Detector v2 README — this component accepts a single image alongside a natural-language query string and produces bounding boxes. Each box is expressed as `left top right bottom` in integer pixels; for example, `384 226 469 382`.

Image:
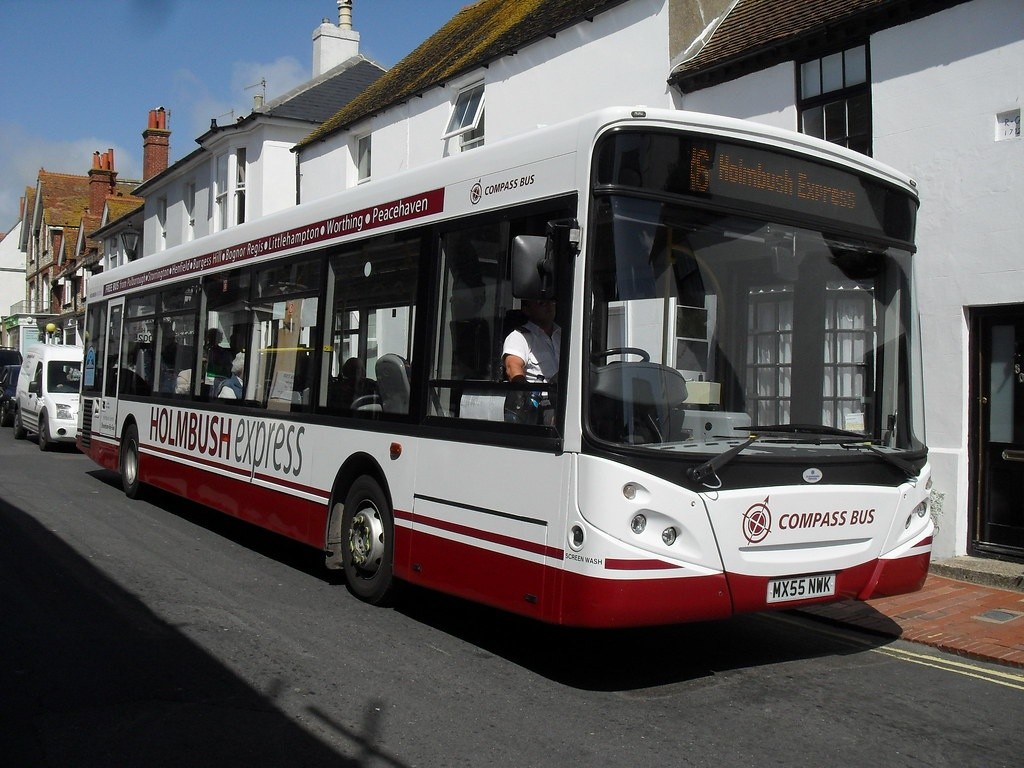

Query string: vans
14 342 86 453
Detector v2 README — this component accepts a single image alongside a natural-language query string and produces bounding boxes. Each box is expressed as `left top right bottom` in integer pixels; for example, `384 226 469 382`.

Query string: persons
337 358 377 405
501 300 562 427
39 364 63 396
175 369 192 394
204 328 223 348
214 352 244 399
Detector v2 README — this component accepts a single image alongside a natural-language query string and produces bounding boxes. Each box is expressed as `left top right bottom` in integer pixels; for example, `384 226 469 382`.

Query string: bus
76 107 939 629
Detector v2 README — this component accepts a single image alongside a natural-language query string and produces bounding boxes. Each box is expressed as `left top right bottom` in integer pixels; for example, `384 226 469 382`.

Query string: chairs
375 353 410 413
502 307 529 339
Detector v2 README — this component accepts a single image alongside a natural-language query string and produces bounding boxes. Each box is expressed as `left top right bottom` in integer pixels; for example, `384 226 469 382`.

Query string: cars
0 364 22 428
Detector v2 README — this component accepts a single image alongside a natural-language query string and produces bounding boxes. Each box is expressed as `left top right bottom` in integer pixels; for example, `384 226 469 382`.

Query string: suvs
0 345 23 377
